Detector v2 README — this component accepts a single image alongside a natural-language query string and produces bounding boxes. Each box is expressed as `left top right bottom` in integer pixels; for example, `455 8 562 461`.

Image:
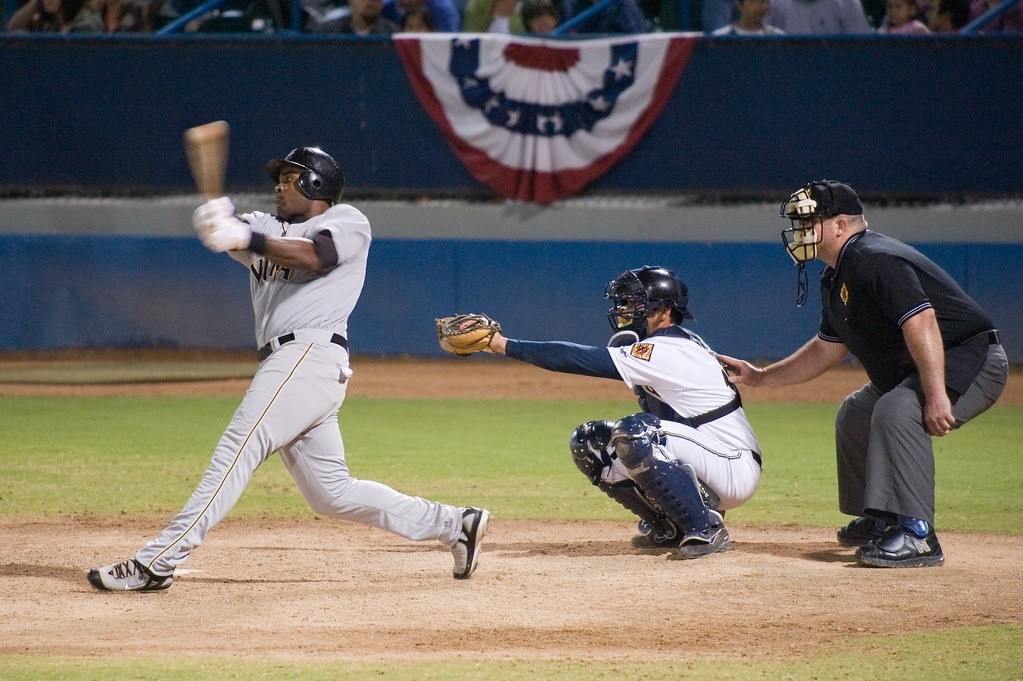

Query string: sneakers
836 516 897 546
681 527 732 559
88 560 174 591
452 508 493 580
631 530 683 548
856 526 947 567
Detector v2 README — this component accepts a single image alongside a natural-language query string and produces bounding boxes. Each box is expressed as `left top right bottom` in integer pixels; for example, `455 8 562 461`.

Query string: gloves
192 194 266 255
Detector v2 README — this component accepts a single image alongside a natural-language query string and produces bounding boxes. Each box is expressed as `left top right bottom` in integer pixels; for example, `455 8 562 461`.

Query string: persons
436 264 763 558
708 180 1008 567
7 0 1023 38
88 146 492 594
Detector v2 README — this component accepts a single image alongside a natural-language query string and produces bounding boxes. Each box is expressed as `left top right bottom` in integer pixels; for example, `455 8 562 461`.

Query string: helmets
267 146 347 205
629 266 694 318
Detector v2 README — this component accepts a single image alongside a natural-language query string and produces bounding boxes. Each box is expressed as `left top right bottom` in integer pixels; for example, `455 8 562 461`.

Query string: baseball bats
181 121 229 206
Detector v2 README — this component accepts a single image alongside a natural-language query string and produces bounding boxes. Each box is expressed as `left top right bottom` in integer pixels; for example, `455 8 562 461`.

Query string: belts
751 450 762 467
989 330 1001 345
257 330 348 362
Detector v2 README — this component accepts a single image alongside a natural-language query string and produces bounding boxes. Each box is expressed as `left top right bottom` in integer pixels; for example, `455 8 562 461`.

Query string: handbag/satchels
941 334 990 396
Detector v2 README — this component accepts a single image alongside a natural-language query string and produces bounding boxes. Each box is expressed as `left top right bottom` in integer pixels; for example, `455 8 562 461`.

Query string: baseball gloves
434 312 503 359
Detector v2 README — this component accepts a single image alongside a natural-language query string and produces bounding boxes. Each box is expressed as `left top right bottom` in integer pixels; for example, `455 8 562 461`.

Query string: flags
394 33 705 205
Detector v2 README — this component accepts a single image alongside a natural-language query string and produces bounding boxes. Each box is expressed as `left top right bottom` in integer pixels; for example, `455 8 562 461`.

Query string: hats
806 181 864 218
